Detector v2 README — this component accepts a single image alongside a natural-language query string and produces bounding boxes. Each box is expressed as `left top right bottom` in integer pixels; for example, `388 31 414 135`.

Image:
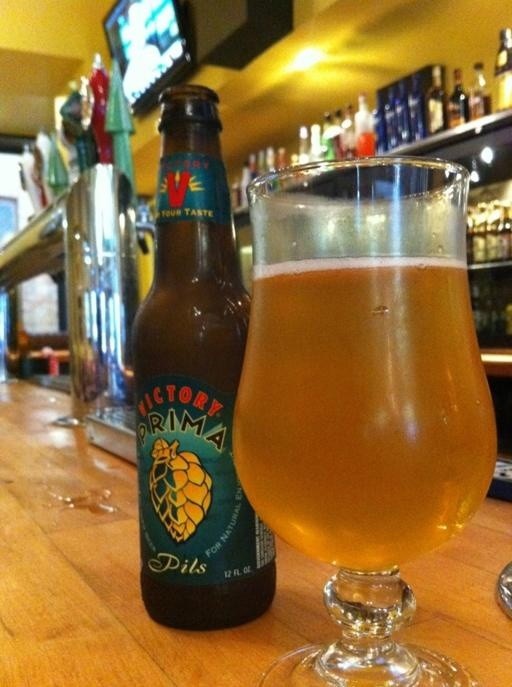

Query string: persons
121 1 163 103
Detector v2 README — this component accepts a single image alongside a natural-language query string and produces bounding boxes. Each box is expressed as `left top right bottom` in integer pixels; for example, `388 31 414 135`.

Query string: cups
60 159 144 425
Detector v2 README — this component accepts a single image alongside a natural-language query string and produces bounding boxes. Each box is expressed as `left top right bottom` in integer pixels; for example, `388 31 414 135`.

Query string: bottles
134 81 272 633
241 28 512 356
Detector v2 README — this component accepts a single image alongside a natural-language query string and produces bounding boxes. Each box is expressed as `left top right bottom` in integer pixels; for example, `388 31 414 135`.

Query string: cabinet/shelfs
232 107 512 376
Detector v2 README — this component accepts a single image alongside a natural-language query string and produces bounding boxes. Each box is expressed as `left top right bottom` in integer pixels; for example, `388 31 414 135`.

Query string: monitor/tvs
101 0 194 119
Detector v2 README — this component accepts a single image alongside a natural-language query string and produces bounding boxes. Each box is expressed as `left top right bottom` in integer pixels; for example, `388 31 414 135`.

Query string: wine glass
229 154 500 687
